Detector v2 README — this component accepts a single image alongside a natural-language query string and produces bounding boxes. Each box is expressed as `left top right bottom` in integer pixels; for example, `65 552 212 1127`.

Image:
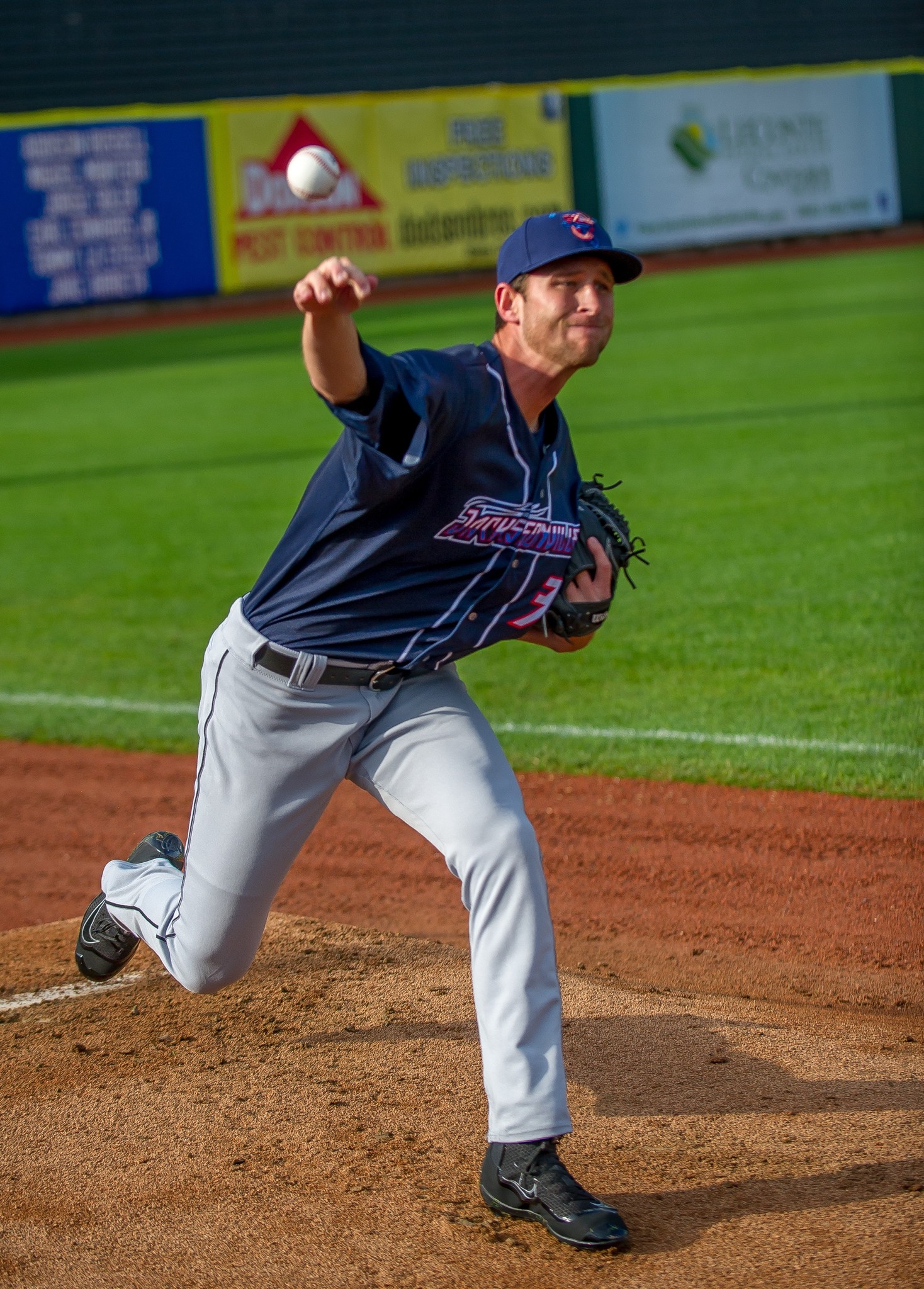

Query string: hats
495 211 643 286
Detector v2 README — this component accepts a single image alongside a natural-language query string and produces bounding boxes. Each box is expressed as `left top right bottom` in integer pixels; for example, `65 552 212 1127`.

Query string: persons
75 209 642 1247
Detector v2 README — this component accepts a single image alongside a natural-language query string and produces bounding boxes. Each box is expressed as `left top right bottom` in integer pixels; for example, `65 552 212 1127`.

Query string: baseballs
286 145 340 203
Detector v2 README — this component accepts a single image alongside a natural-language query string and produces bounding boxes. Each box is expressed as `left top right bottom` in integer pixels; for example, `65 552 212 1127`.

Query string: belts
253 644 408 693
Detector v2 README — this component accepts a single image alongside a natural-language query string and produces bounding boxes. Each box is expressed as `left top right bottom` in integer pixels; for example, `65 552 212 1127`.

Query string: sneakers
73 831 186 982
478 1138 628 1248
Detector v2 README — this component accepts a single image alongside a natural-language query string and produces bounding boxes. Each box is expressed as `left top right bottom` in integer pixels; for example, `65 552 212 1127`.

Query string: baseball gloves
530 480 634 639
